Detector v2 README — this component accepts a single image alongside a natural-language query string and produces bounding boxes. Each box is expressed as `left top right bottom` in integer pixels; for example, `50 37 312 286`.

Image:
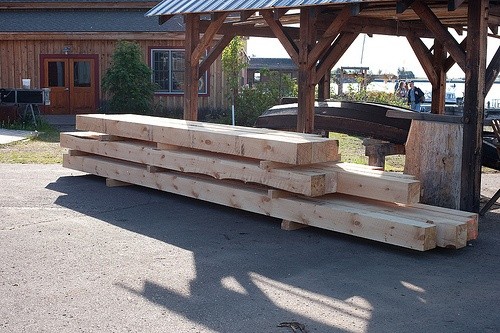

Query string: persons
404 82 410 97
396 81 406 97
408 80 424 111
